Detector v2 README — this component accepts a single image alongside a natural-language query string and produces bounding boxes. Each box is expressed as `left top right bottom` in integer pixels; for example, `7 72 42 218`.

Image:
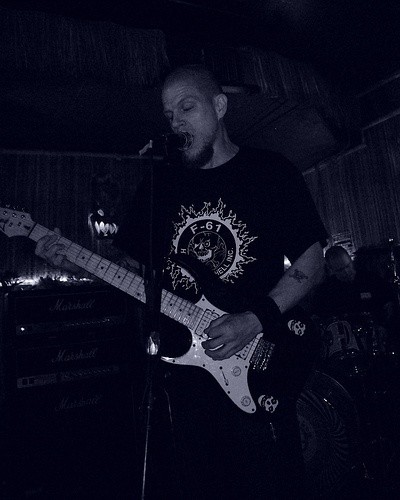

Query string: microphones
160 132 191 152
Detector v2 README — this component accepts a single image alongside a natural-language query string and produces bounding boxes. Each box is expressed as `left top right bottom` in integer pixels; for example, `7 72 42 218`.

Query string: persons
316 246 393 340
35 64 330 500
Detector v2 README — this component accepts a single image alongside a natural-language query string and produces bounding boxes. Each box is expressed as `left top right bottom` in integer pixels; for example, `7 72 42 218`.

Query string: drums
302 371 362 496
361 335 390 372
318 316 361 370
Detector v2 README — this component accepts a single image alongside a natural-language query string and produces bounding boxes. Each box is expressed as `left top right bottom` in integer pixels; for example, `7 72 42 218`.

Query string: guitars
0 204 315 423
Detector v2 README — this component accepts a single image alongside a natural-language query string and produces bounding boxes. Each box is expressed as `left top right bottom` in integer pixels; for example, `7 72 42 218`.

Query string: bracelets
245 292 282 334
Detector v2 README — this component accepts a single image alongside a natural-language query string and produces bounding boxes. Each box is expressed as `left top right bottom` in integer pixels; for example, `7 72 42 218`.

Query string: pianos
11 315 139 360
